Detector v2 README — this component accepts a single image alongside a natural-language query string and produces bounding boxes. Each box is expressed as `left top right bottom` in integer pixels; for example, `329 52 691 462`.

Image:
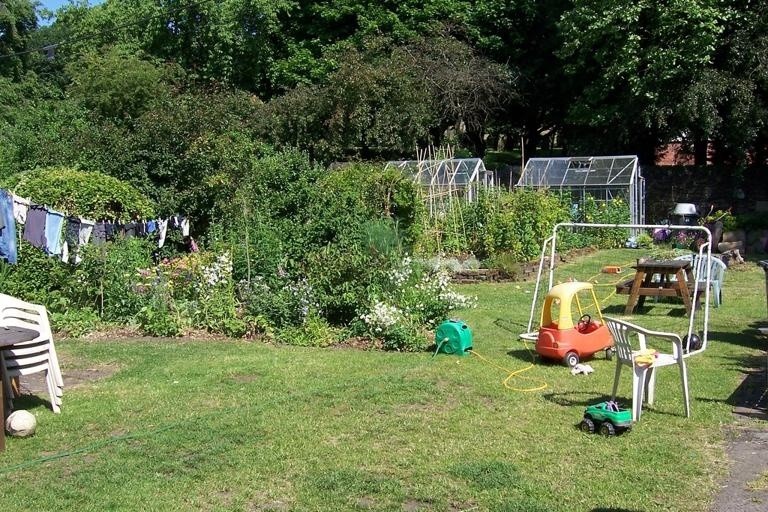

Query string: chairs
600 315 690 421
651 254 728 309
1 291 65 418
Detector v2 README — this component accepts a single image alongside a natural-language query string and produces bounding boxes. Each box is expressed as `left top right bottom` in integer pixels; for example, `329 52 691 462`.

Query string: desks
624 260 703 319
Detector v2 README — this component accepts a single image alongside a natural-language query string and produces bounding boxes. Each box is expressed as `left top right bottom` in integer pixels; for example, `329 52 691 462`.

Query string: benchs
615 278 712 314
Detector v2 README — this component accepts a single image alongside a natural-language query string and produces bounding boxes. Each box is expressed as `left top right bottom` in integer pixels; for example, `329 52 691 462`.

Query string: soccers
683 333 701 350
